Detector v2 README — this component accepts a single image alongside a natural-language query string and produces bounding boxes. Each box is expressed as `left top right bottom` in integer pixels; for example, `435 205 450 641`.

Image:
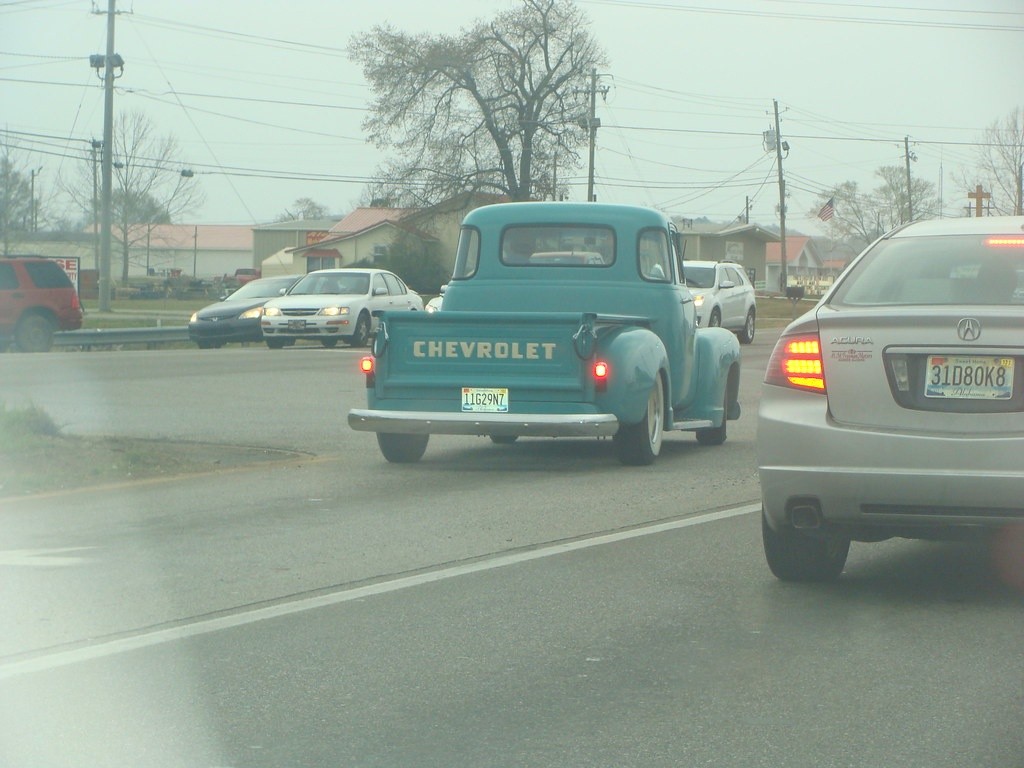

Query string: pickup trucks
347 201 742 467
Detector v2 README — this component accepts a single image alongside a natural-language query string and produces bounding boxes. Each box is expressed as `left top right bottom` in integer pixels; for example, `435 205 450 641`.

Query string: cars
262 267 423 349
188 274 304 347
424 284 448 313
755 215 1024 589
1 251 85 353
231 268 260 290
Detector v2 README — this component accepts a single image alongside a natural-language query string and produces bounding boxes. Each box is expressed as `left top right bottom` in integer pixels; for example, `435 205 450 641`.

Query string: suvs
667 259 759 344
